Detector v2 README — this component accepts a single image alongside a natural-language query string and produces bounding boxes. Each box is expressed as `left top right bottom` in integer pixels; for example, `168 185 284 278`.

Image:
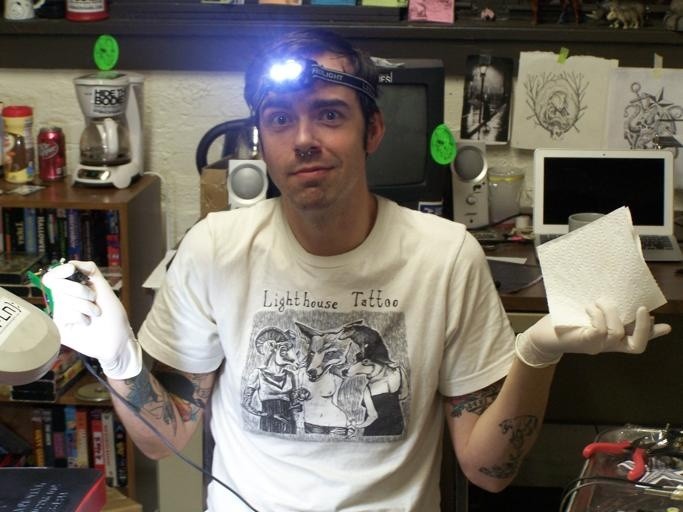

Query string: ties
269 57 447 219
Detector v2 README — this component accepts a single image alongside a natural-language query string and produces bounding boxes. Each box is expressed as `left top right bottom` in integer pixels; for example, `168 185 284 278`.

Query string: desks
141 208 682 512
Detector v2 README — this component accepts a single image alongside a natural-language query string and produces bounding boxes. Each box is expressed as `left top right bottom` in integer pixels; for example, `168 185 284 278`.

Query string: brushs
72 69 146 191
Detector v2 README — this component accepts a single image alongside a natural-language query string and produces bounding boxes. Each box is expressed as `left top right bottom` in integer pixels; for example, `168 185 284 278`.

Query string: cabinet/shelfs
0 173 164 512
0 104 35 183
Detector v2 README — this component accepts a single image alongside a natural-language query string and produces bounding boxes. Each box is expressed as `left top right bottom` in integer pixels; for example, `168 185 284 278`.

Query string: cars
568 212 604 233
487 161 526 225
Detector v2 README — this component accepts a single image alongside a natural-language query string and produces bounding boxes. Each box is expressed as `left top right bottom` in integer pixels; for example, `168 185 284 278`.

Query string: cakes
38 127 65 182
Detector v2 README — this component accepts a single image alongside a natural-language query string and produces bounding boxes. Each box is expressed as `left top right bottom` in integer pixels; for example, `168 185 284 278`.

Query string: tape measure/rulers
582 436 656 480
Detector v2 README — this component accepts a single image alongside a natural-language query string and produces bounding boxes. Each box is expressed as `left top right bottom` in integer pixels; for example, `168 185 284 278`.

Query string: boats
451 139 490 230
227 158 270 210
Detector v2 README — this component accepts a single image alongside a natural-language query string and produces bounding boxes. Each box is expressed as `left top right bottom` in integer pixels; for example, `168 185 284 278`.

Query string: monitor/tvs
0 208 129 494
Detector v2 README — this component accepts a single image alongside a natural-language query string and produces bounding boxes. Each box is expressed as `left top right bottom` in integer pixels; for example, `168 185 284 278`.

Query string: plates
0 173 164 512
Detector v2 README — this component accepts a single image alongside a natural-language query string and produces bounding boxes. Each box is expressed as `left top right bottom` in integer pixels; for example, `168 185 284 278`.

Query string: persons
37 25 674 512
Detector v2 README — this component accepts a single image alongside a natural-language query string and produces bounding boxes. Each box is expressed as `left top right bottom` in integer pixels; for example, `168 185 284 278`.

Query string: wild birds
533 149 682 263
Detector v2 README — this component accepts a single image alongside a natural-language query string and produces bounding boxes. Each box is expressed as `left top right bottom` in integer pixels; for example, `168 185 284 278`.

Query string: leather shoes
514 301 672 369
41 259 144 380
